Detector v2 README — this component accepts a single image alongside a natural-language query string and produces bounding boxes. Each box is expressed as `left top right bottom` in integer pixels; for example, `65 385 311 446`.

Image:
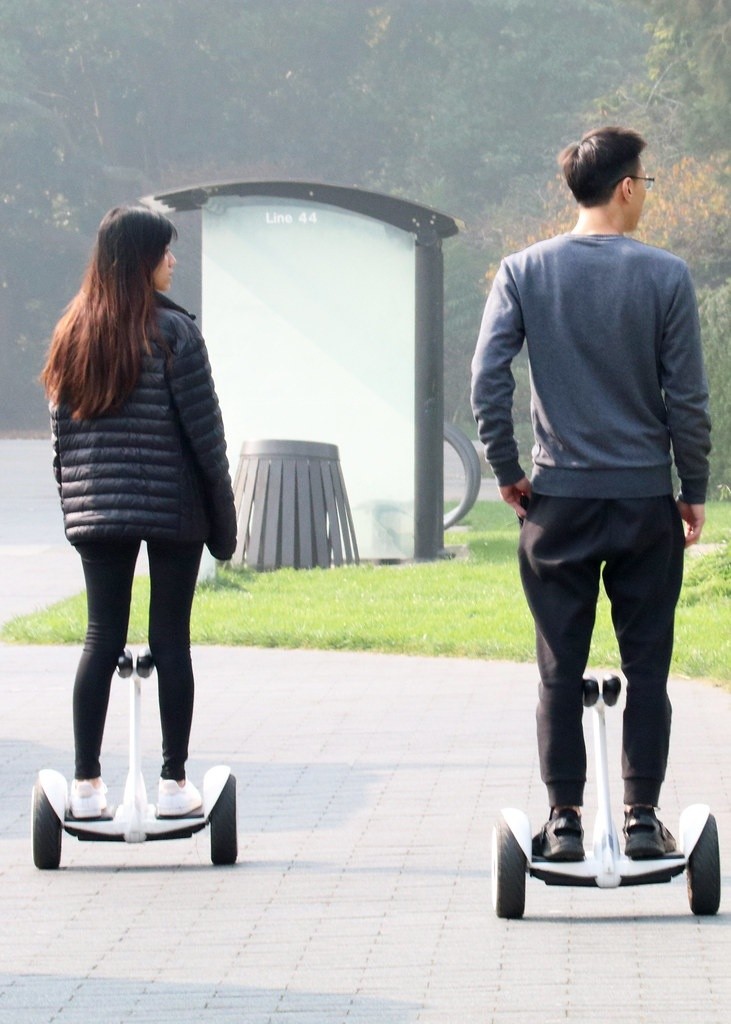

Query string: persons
467 125 716 858
39 204 239 821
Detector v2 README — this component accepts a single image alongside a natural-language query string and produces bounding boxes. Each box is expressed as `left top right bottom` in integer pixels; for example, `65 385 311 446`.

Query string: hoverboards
29 650 237 866
495 674 720 921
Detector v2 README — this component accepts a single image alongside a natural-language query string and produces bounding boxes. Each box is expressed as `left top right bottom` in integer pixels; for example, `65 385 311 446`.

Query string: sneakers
70 778 108 817
532 809 585 861
622 805 677 856
158 777 203 815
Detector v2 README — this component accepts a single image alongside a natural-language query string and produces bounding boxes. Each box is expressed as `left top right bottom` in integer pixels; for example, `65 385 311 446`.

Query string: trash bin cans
221 439 360 572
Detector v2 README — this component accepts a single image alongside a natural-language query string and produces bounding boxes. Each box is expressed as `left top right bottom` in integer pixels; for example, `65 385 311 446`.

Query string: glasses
618 174 655 191
